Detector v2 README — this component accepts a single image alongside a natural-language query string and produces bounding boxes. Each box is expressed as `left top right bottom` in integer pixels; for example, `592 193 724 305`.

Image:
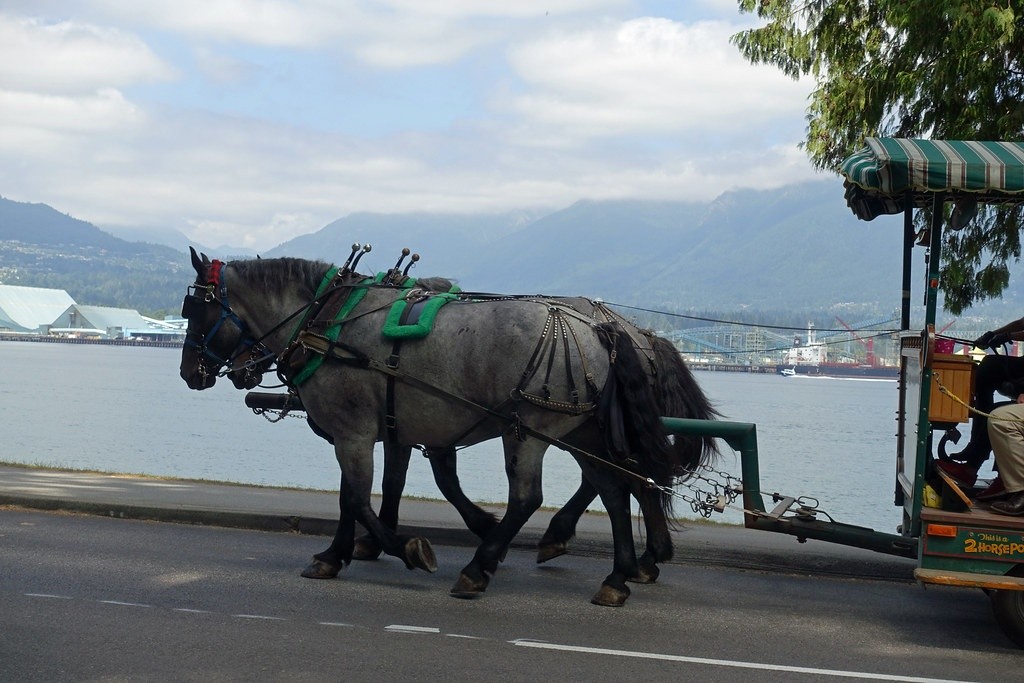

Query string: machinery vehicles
832 313 879 370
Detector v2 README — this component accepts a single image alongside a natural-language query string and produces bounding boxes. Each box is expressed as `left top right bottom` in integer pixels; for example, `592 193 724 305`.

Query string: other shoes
949 442 981 461
974 477 1007 498
990 493 1024 515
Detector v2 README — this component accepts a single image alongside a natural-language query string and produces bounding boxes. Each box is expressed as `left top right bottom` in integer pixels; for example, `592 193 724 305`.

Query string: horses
180 245 721 607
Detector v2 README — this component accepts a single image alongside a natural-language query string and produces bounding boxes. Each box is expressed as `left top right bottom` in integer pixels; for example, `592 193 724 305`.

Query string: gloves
972 330 994 350
988 331 1013 348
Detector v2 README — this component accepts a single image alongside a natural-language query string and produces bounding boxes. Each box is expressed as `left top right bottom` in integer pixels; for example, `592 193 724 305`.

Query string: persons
935 318 1024 516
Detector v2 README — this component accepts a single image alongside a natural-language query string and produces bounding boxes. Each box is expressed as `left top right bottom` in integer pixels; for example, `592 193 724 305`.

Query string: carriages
176 136 1024 647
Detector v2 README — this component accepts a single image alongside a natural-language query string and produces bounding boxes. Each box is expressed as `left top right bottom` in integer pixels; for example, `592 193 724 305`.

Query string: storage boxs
928 353 978 423
